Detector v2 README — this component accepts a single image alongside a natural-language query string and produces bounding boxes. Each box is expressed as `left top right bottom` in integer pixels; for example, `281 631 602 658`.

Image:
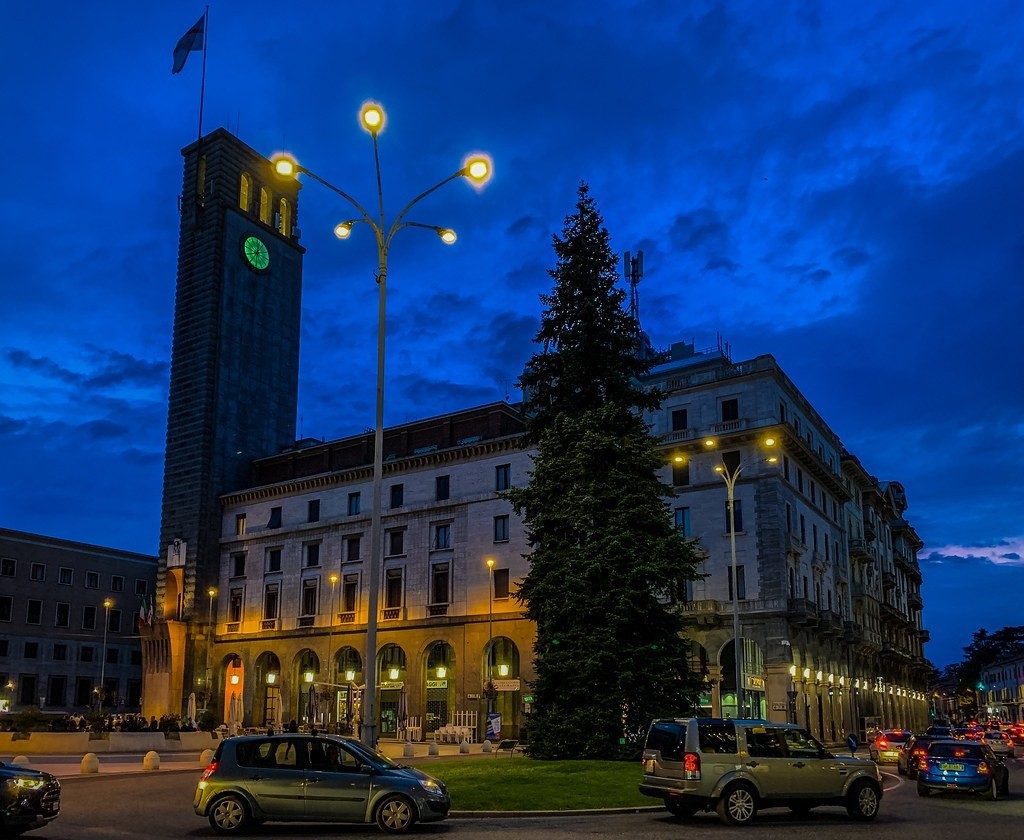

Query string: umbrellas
187 690 196 722
274 694 282 734
345 682 355 734
228 691 236 735
237 693 244 729
306 683 317 720
396 686 408 740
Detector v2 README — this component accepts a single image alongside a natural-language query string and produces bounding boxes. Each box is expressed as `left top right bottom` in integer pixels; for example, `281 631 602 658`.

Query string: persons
307 720 352 735
320 745 341 772
268 725 275 736
47 697 228 733
289 720 298 733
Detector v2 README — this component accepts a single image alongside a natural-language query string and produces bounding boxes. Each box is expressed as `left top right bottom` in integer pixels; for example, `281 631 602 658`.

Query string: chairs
434 726 471 744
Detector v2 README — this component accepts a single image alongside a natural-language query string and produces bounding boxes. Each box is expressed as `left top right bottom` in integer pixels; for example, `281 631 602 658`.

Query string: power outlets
266 655 276 684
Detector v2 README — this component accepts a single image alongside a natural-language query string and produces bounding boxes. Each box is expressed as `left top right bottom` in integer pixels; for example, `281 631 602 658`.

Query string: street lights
203 591 215 708
97 602 112 712
265 100 495 747
486 559 494 684
327 575 338 690
669 433 783 719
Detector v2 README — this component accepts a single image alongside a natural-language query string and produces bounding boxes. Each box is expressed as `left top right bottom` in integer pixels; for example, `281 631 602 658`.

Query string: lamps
198 668 211 685
496 637 510 676
388 644 399 679
302 650 314 682
435 640 447 677
229 656 239 685
345 646 355 681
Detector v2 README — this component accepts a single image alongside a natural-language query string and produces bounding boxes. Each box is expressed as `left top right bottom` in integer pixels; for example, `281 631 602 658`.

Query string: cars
0 761 63 840
192 731 452 836
868 729 914 766
916 738 1009 802
896 709 1024 780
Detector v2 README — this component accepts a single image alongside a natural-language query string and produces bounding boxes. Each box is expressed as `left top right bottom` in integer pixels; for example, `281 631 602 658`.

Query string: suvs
637 717 884 827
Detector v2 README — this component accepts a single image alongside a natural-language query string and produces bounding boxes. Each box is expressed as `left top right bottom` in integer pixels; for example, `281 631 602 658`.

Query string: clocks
239 232 273 276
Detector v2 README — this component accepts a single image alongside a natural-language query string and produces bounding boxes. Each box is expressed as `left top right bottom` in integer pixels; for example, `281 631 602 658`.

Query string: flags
172 14 205 74
138 599 148 628
147 596 153 627
